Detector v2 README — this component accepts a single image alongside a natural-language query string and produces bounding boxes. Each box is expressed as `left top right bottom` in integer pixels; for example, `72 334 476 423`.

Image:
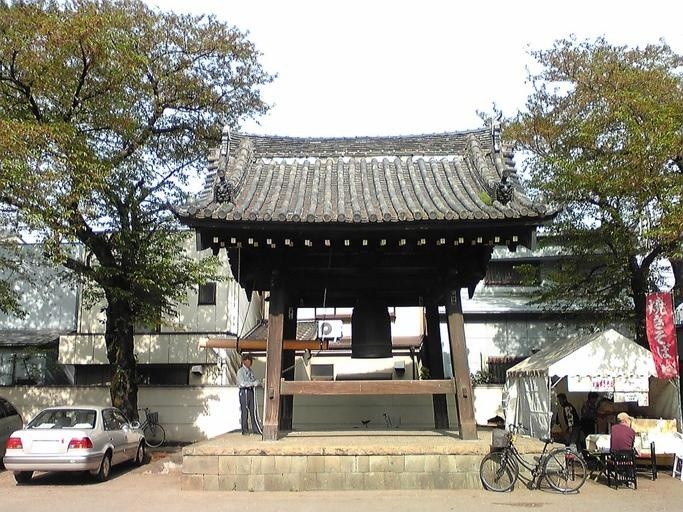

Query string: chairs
606 448 637 490
77 414 95 426
55 417 71 427
636 441 657 481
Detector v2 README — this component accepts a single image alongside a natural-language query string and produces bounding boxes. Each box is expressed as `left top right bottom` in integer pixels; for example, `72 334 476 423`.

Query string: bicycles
137 406 165 449
351 411 402 430
480 422 589 496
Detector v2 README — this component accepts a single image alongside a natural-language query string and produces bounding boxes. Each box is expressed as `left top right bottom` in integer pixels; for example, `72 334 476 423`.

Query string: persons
572 391 627 470
610 412 635 488
237 354 262 435
552 393 580 446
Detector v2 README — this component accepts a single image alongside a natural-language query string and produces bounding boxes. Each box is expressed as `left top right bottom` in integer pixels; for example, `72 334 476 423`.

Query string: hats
617 413 633 422
242 354 255 361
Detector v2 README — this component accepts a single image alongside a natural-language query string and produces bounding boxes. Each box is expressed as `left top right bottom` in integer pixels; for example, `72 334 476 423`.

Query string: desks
583 449 637 482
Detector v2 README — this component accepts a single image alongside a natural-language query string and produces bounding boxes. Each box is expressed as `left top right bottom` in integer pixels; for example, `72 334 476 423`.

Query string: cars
2 404 147 482
0 395 23 460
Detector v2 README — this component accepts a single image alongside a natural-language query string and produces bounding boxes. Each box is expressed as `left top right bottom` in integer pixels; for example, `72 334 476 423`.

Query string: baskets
492 428 510 448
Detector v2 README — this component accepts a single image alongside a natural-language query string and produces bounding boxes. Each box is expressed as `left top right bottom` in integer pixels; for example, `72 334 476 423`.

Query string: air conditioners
318 320 343 338
